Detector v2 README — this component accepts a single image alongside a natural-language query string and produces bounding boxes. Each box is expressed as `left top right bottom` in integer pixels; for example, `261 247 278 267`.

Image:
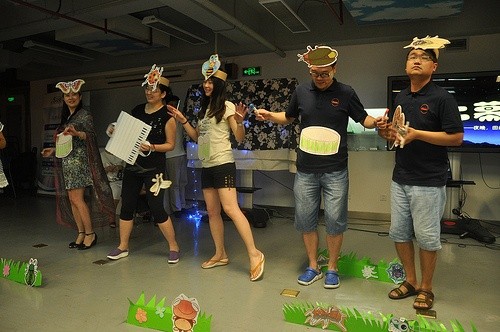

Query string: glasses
407 53 437 63
310 68 332 77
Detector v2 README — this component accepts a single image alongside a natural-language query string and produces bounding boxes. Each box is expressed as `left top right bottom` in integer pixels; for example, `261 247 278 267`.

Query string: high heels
69 232 85 248
77 232 97 250
250 253 265 281
202 258 229 268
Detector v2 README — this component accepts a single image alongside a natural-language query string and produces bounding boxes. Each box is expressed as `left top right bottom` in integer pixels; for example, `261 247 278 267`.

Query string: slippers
388 281 420 299
297 268 323 286
325 271 340 288
413 290 434 309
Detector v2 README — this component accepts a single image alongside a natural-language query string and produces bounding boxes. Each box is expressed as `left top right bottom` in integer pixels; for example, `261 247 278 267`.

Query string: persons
378 49 464 310
105 77 180 263
166 67 265 281
0 121 9 188
254 43 389 288
41 90 116 250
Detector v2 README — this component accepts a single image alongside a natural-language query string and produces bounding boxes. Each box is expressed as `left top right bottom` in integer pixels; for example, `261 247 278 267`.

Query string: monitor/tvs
387 70 500 153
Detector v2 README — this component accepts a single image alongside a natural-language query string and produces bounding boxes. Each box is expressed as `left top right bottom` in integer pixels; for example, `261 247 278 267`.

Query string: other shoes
107 248 128 259
167 250 180 264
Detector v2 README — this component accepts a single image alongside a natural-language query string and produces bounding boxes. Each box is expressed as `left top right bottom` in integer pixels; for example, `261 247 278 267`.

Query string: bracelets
182 121 187 125
